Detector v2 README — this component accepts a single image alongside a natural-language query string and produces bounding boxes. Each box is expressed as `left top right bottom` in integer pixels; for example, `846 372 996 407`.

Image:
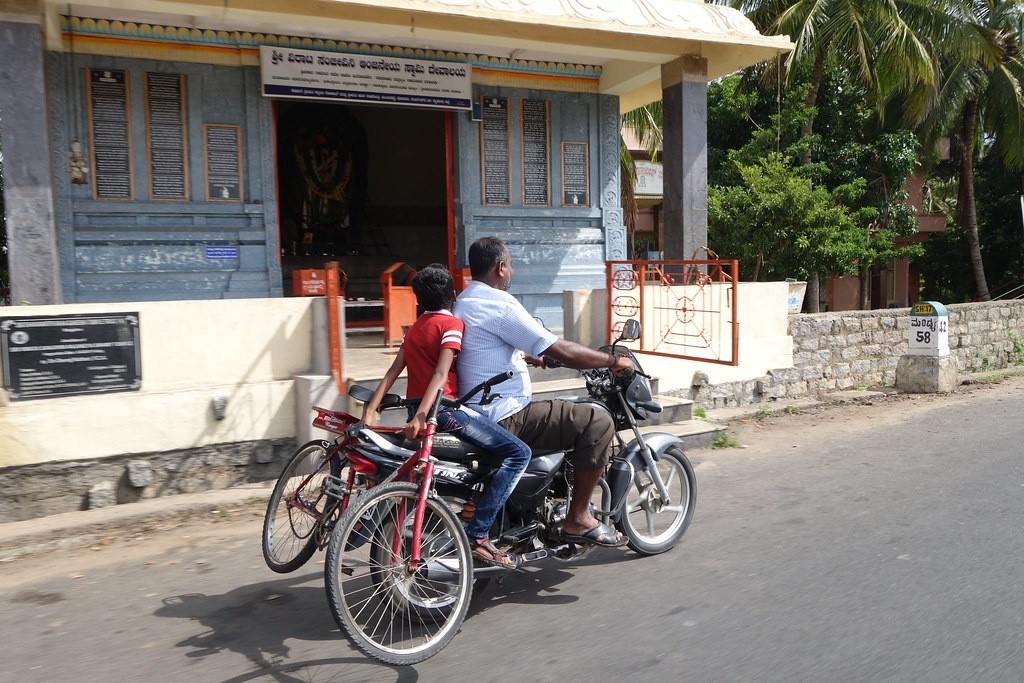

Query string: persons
450 236 637 548
351 262 532 571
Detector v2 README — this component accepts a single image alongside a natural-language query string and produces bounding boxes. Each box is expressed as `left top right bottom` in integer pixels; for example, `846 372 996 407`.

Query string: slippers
563 517 629 546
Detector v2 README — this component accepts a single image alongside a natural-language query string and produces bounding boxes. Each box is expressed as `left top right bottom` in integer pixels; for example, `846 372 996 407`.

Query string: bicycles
262 370 515 665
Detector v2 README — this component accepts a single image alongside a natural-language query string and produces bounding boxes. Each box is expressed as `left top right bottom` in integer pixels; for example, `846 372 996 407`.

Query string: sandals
464 530 516 569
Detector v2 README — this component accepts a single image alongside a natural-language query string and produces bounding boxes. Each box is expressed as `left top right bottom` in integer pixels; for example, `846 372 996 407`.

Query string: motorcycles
340 318 698 625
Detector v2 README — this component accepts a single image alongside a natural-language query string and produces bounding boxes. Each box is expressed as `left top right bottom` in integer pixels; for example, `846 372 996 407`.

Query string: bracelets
611 355 617 368
